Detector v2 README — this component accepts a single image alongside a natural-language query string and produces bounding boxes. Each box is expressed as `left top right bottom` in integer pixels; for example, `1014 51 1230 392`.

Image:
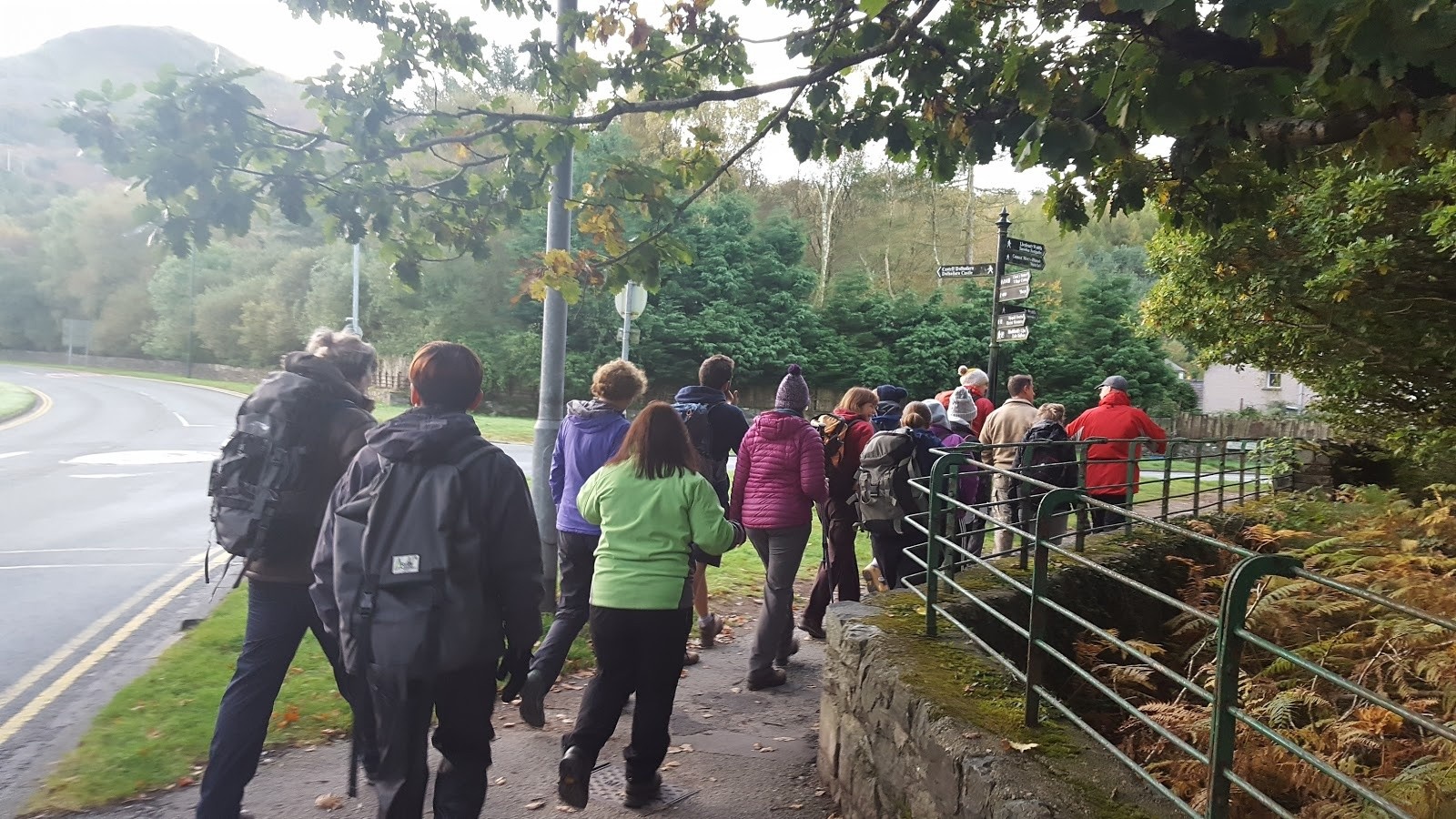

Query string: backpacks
810 412 862 477
855 429 916 535
205 371 325 559
1022 431 1071 506
935 433 979 510
333 433 505 698
667 400 715 486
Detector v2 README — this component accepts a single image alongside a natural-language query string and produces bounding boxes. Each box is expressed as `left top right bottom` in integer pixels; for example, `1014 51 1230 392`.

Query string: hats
921 399 952 431
946 387 978 421
775 364 810 410
958 365 990 386
1093 376 1127 393
876 385 907 402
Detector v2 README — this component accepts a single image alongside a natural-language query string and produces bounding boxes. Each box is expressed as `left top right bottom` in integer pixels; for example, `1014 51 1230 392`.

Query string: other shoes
519 669 552 727
697 614 724 648
797 617 826 639
683 651 699 666
558 745 594 809
747 667 788 691
622 772 665 808
776 639 800 666
862 564 886 593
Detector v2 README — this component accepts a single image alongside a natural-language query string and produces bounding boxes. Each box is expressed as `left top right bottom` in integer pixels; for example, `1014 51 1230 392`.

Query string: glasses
1098 386 1112 391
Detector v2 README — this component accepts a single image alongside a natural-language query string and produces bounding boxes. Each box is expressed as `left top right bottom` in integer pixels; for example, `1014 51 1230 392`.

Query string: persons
310 340 544 819
193 333 377 819
517 362 1172 810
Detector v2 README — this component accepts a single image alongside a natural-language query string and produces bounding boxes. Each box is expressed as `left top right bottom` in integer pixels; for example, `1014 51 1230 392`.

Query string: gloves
728 518 747 546
497 654 530 703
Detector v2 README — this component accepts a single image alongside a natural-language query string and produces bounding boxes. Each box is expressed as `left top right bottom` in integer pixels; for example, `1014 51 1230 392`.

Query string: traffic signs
1000 304 1041 321
998 269 1032 303
937 263 995 278
994 311 1031 344
1003 238 1046 270
